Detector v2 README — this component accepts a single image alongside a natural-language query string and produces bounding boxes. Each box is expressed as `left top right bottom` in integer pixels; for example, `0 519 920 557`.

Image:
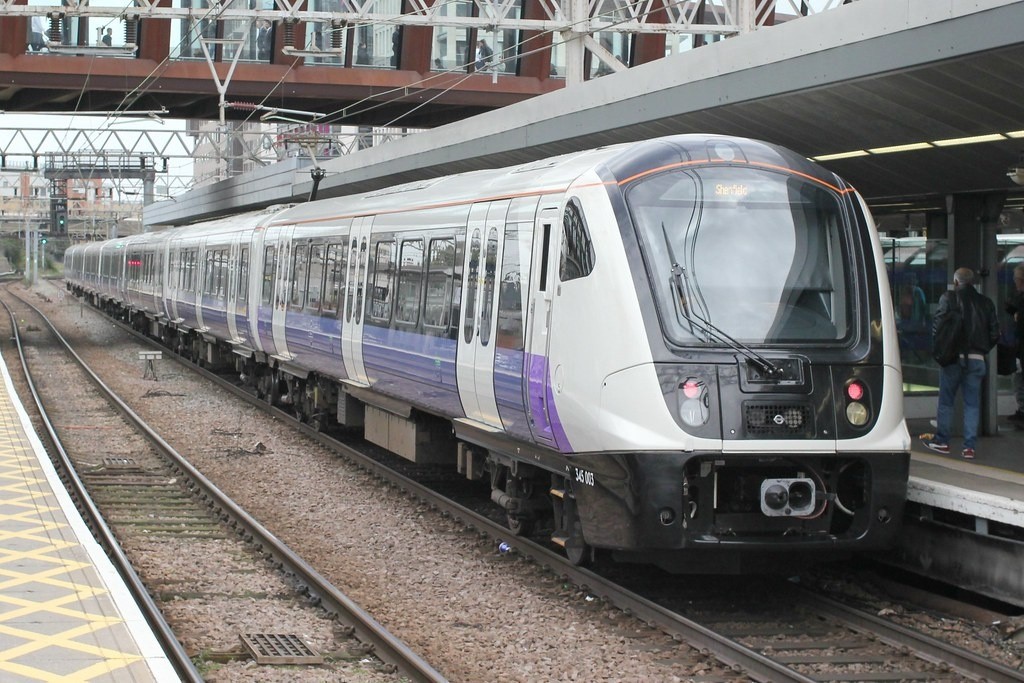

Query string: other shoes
1008 411 1024 420
1016 422 1024 430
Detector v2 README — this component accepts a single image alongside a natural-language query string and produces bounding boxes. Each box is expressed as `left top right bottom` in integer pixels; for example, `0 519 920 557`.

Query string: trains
62 132 910 587
876 235 1024 368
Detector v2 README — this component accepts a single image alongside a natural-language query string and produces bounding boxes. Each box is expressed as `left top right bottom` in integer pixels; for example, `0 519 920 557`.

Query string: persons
256 19 272 60
354 24 622 77
102 28 112 45
915 267 1001 460
1004 264 1024 429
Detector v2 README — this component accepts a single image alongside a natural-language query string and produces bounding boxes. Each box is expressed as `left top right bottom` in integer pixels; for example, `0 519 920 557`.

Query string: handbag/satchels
929 290 968 366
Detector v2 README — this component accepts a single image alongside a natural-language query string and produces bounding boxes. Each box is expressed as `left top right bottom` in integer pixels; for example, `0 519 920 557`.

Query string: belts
958 354 986 362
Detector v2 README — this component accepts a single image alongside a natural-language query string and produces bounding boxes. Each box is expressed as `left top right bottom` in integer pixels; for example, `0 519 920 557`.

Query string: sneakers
961 448 975 458
919 433 950 454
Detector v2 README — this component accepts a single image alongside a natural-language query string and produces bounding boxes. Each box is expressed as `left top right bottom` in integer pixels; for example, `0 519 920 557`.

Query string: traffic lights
42 237 48 244
58 213 68 234
